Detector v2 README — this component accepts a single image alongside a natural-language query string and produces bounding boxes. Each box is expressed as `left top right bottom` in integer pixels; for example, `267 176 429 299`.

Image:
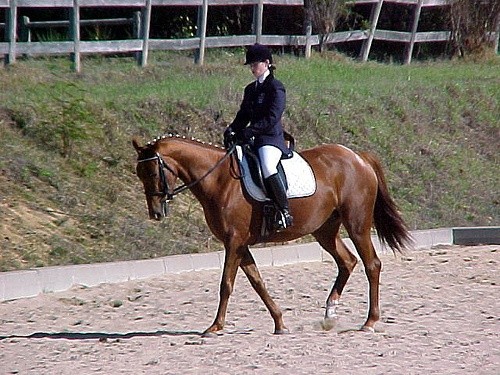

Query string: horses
131 133 415 338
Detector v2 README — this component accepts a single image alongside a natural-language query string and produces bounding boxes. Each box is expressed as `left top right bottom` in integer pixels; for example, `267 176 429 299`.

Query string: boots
263 172 293 229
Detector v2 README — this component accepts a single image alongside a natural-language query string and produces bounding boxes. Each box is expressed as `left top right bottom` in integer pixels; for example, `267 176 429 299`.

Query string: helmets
243 42 273 66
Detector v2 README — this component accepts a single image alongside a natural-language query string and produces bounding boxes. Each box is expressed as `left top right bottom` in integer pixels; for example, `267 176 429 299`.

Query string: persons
224 43 294 229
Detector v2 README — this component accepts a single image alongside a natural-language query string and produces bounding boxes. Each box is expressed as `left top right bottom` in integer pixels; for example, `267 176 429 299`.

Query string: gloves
233 127 254 146
223 128 233 150
